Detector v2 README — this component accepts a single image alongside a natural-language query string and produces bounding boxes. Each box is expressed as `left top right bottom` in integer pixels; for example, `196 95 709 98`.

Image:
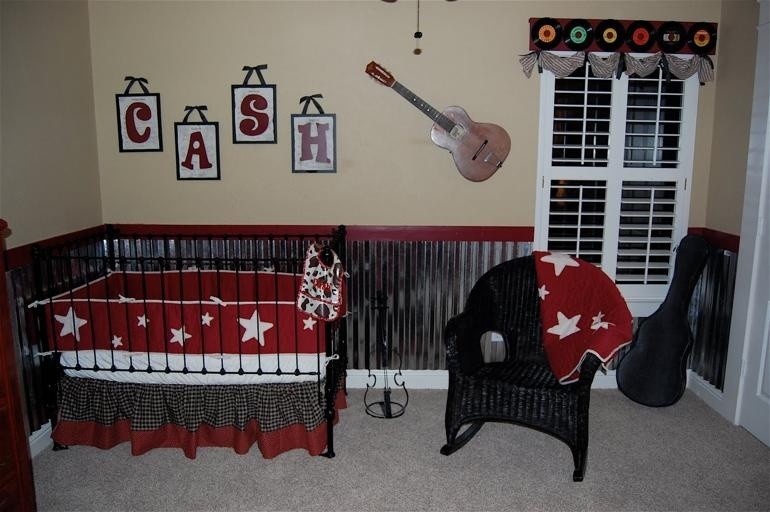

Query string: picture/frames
291 112 338 175
114 91 159 152
230 83 277 144
172 120 221 181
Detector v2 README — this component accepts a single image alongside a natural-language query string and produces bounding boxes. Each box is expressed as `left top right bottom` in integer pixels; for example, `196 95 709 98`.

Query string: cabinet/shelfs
0 218 40 512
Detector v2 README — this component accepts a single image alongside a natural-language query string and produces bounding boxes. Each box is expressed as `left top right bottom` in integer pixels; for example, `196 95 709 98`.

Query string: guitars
364 59 511 182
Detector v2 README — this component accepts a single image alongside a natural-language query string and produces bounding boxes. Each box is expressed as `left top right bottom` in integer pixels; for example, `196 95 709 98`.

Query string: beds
19 218 349 463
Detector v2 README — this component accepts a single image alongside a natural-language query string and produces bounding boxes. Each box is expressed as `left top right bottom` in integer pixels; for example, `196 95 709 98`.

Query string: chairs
438 251 630 482
